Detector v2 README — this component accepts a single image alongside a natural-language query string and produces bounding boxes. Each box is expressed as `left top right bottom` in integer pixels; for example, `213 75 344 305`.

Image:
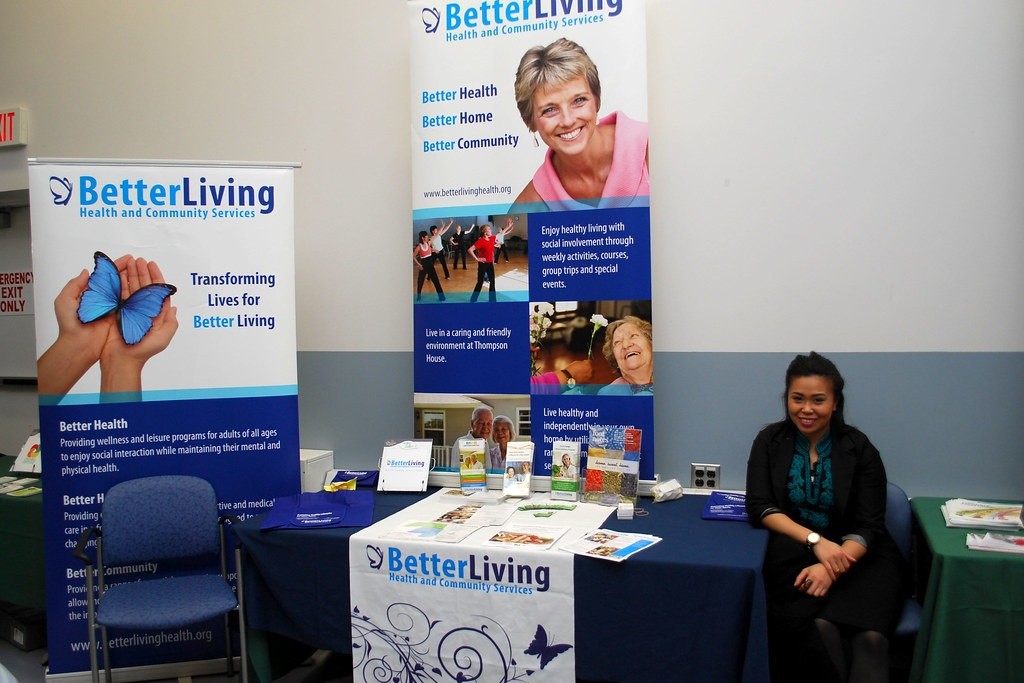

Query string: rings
803 580 812 588
835 569 839 572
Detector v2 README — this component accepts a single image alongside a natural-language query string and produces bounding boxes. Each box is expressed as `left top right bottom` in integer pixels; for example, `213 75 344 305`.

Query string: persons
468 219 514 303
507 462 530 482
36 255 179 405
413 219 454 302
597 315 655 395
450 223 476 269
489 415 516 470
560 454 577 479
745 351 909 683
530 360 594 395
450 406 493 474
508 38 649 215
471 453 483 469
460 457 472 470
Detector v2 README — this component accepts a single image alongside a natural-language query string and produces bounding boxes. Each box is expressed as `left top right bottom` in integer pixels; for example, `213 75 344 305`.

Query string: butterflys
75 250 177 346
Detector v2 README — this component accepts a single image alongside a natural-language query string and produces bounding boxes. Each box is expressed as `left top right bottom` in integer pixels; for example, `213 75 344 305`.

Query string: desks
907 496 1024 683
231 485 770 683
0 454 46 613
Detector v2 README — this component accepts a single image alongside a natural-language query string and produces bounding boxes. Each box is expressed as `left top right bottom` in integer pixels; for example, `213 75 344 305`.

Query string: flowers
588 313 609 360
529 302 555 375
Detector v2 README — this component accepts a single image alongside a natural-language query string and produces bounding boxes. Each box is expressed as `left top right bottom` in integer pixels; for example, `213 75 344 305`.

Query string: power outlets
690 463 721 490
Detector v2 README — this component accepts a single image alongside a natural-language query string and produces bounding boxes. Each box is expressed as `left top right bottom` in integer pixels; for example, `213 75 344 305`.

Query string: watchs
561 370 576 389
806 531 822 549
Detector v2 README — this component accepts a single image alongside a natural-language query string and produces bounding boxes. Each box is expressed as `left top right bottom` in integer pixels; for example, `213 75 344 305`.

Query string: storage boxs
0 601 47 652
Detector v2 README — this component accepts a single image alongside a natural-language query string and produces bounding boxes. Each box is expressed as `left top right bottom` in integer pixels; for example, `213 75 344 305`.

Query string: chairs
442 240 456 260
73 474 248 683
885 483 923 638
